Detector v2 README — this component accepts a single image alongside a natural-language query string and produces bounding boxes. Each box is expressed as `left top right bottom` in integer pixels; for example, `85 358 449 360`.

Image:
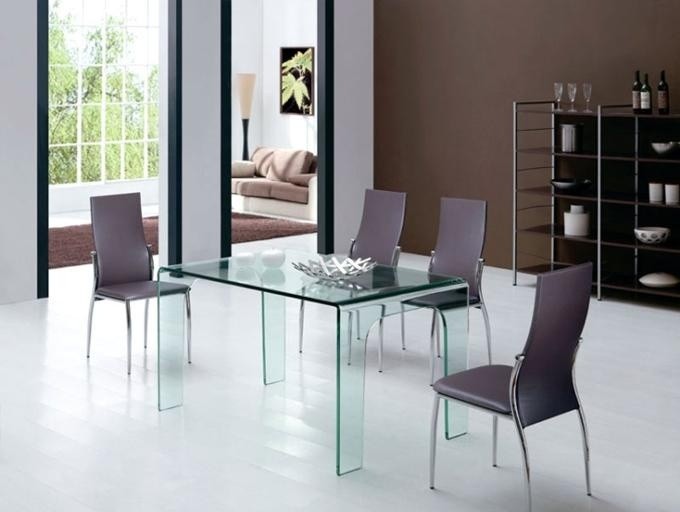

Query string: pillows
288 174 316 186
266 150 313 182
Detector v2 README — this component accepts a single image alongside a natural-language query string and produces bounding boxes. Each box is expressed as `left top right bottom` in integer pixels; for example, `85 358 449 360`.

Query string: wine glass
552 81 592 114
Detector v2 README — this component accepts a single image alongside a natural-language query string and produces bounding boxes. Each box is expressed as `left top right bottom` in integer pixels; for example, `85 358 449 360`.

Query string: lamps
234 71 258 162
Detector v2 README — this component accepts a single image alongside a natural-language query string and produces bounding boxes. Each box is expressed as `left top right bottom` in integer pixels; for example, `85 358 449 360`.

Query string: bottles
630 69 670 115
563 204 591 237
559 123 577 153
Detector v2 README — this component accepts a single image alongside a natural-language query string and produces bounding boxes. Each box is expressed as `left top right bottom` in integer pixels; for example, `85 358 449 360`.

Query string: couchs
231 147 317 226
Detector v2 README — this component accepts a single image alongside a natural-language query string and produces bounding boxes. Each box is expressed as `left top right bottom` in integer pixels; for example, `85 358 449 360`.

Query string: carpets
48 210 318 269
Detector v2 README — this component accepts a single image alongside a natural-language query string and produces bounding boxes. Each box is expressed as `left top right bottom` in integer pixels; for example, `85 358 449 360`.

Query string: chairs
422 259 601 512
296 186 410 375
398 194 496 387
80 188 196 376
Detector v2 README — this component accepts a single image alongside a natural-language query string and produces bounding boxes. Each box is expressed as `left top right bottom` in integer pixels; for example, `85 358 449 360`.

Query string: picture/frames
279 46 315 115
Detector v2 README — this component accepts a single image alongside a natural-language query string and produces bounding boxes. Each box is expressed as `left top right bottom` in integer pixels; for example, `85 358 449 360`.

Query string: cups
648 182 662 202
664 183 679 204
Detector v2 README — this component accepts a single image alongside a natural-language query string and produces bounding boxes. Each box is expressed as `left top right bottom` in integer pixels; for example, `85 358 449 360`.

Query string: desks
148 249 472 475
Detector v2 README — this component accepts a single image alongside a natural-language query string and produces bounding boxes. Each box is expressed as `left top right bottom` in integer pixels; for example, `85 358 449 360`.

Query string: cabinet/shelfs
516 96 679 304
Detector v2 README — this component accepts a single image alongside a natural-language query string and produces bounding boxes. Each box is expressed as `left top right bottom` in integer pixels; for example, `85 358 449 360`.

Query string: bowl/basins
550 178 592 197
651 142 680 154
634 226 670 244
